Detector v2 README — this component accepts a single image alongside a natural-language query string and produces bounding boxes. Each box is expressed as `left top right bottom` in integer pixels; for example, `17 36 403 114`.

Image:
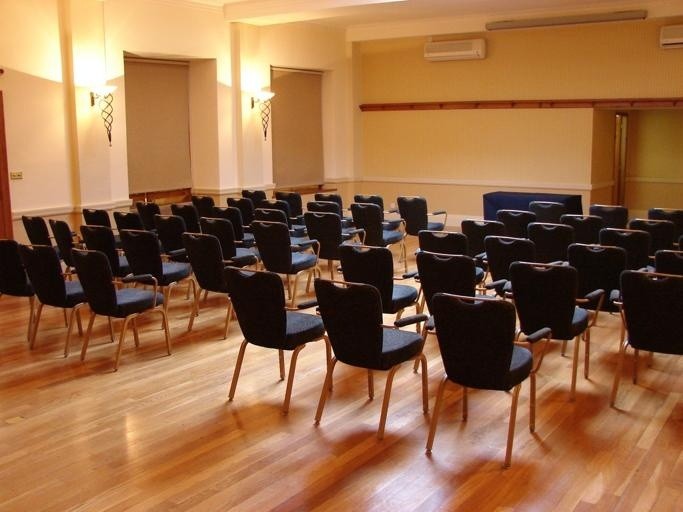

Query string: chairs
20 245 115 359
70 248 172 369
315 274 429 441
2 239 68 341
337 199 683 409
424 292 552 467
22 190 449 340
225 265 334 416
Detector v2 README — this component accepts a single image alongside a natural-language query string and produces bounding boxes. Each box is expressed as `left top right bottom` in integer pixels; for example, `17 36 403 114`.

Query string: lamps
251 90 276 140
90 85 118 146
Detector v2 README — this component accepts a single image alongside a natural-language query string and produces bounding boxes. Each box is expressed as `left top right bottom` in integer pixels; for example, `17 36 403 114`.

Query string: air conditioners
659 24 683 48
423 37 486 61
